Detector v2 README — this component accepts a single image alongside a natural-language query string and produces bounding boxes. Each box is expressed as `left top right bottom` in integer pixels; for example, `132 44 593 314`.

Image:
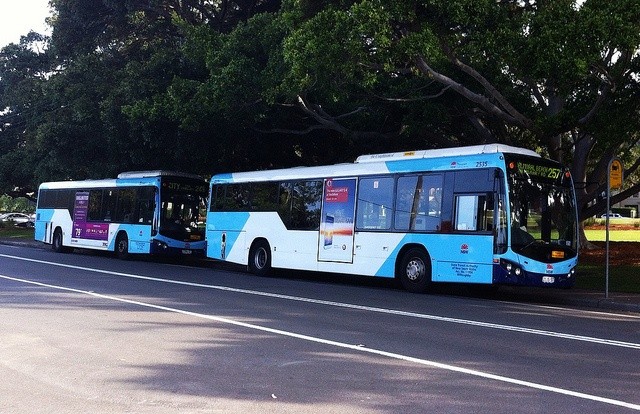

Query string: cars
601 212 627 218
1 213 35 227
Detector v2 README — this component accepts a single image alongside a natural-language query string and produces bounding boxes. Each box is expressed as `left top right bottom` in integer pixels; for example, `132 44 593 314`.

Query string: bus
35 170 211 261
203 144 579 294
